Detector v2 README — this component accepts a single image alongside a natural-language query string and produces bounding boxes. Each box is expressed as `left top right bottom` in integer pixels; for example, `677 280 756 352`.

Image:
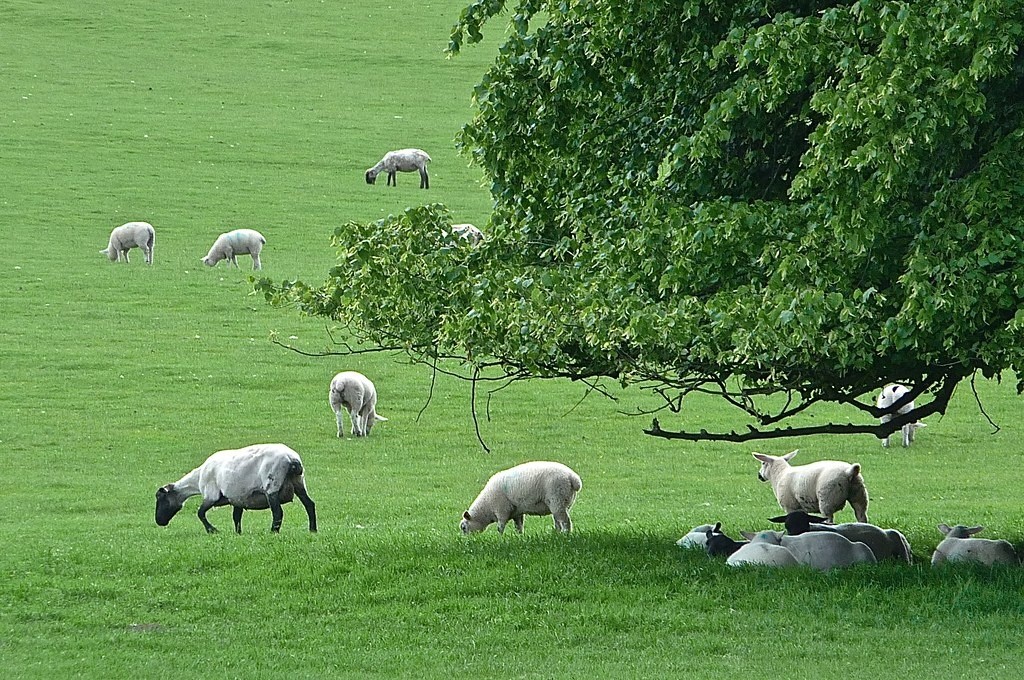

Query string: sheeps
155 443 318 535
876 384 927 448
672 510 1022 570
751 449 869 525
365 148 432 190
99 221 156 266
199 229 266 271
429 223 486 251
328 370 389 437
458 460 582 535
